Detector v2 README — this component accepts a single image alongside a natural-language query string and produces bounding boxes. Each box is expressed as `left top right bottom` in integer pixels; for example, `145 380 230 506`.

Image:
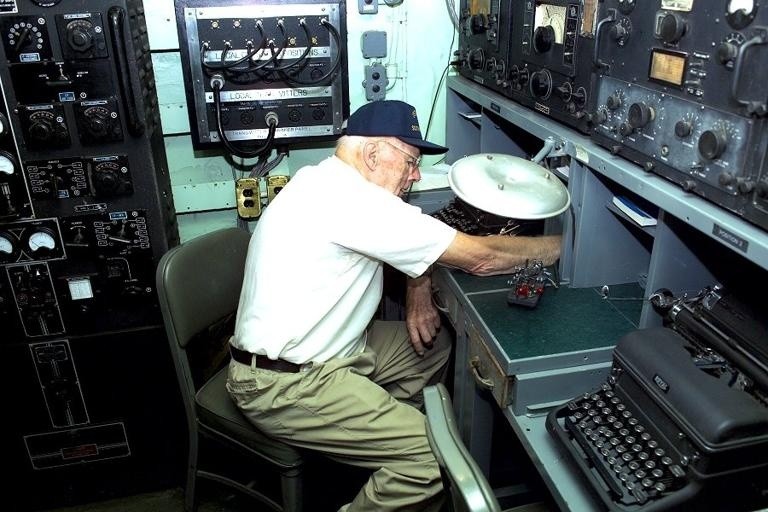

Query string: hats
340 97 449 156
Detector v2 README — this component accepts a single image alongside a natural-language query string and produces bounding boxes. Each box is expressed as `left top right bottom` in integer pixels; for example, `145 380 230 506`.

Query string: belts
227 339 303 376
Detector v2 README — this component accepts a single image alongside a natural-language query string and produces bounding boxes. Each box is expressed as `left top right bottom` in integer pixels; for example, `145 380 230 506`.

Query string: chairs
156 226 312 512
423 382 546 512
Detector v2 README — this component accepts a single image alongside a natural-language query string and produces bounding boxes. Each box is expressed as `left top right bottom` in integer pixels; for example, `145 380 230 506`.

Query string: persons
225 99 566 511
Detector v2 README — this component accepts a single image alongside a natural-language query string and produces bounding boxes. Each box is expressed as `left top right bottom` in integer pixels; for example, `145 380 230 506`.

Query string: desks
380 190 645 512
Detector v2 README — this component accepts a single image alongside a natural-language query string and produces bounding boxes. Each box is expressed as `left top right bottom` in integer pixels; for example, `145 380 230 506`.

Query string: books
611 196 659 228
